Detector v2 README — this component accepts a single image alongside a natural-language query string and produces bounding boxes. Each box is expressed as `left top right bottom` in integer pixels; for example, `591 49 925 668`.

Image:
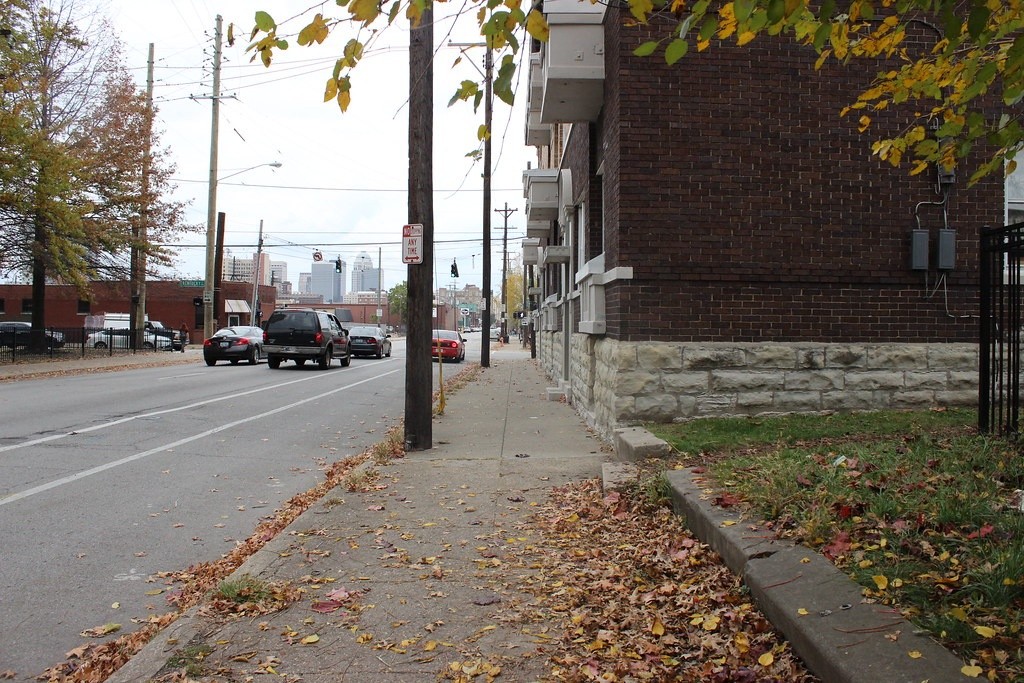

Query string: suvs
145 320 190 350
262 307 352 370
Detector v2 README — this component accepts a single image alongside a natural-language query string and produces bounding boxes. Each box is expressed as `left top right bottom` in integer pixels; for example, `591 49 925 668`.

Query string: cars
433 329 467 363
204 326 268 365
87 327 173 350
348 326 391 357
2 322 65 351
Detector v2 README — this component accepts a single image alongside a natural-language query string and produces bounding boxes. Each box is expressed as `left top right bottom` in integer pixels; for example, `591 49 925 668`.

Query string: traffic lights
336 259 342 274
451 262 459 279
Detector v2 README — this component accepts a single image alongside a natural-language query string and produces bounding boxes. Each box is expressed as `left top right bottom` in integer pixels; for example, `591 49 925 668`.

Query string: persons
179 323 190 353
